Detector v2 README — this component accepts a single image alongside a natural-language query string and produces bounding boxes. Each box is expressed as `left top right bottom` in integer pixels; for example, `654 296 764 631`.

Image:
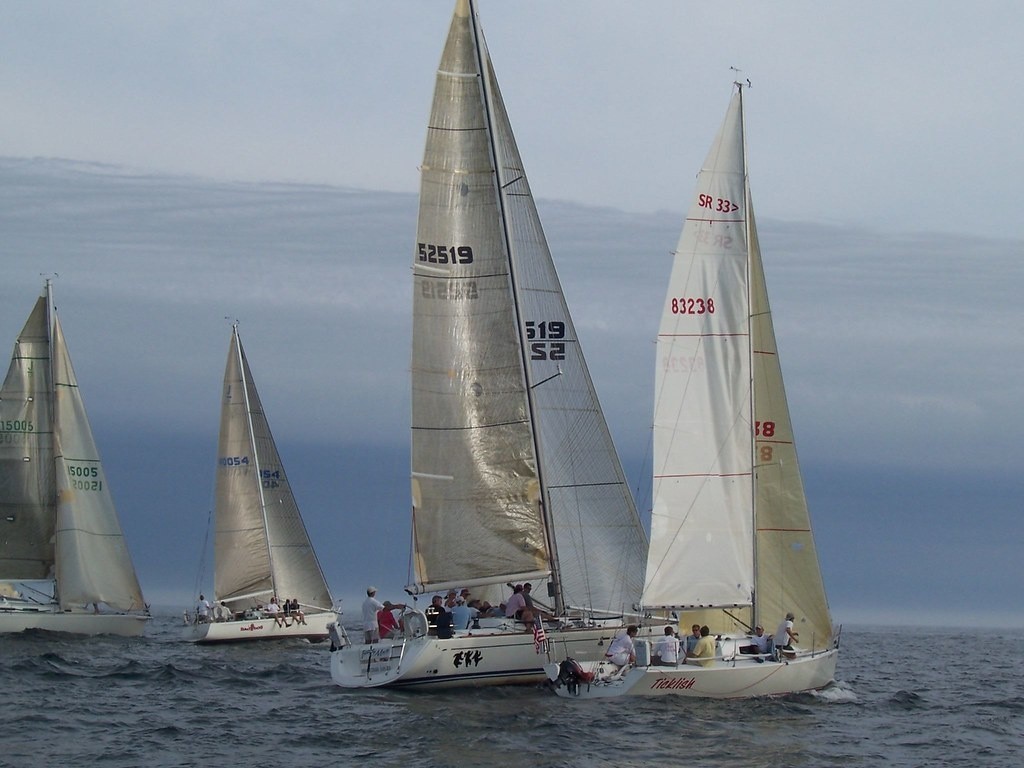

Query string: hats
756 625 763 629
514 585 523 590
785 613 793 620
449 589 458 593
367 587 378 594
461 589 471 595
456 596 464 601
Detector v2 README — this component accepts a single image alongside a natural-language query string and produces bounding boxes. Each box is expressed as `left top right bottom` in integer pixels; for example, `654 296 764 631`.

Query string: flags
532 614 545 655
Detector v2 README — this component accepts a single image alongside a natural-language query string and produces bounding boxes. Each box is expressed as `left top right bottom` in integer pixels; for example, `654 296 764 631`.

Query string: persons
363 587 399 644
197 594 233 622
652 626 679 667
267 598 307 628
605 624 638 665
681 624 715 667
424 582 535 639
750 612 799 664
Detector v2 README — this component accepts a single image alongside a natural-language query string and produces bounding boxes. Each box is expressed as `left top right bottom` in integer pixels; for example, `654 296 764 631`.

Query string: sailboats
329 0 661 690
180 321 342 642
0 280 154 636
543 71 840 699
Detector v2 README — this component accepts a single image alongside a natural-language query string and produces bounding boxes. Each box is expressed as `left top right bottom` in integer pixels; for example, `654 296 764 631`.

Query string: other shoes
280 624 281 628
302 622 307 625
286 624 291 627
297 621 301 625
